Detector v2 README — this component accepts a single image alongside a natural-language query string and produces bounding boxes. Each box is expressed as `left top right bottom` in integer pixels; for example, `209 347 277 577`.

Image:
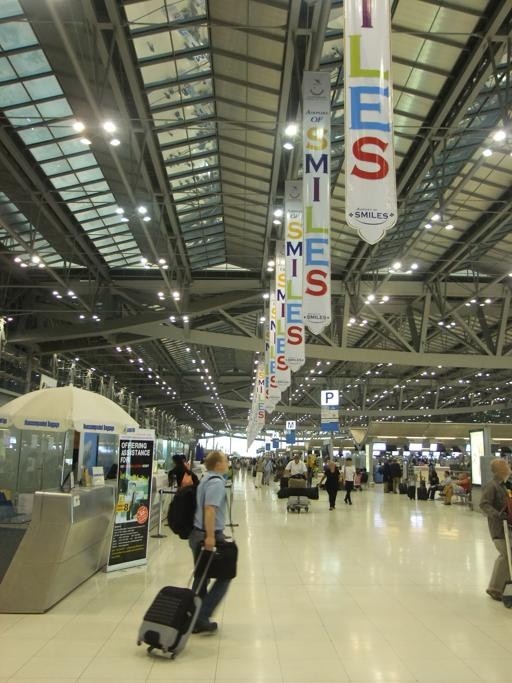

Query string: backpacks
167 472 224 542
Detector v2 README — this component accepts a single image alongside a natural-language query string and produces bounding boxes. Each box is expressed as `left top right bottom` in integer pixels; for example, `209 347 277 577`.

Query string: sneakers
191 620 220 637
328 497 353 511
485 589 503 602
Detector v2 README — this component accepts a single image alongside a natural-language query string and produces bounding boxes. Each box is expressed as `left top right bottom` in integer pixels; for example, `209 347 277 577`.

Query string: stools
453 487 472 506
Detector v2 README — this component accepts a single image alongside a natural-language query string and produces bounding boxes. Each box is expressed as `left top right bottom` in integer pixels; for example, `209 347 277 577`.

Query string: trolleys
284 470 313 516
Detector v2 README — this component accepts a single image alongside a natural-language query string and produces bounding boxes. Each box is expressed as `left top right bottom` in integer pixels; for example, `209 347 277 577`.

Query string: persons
478 457 512 601
172 454 187 490
180 454 190 470
188 450 235 634
240 453 471 515
127 482 139 519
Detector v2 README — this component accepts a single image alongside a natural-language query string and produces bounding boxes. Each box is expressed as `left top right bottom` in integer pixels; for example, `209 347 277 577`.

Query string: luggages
398 477 429 500
287 495 312 507
136 546 217 661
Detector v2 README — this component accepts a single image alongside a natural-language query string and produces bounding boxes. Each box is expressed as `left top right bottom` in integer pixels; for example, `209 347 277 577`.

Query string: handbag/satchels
287 476 308 489
194 536 240 581
